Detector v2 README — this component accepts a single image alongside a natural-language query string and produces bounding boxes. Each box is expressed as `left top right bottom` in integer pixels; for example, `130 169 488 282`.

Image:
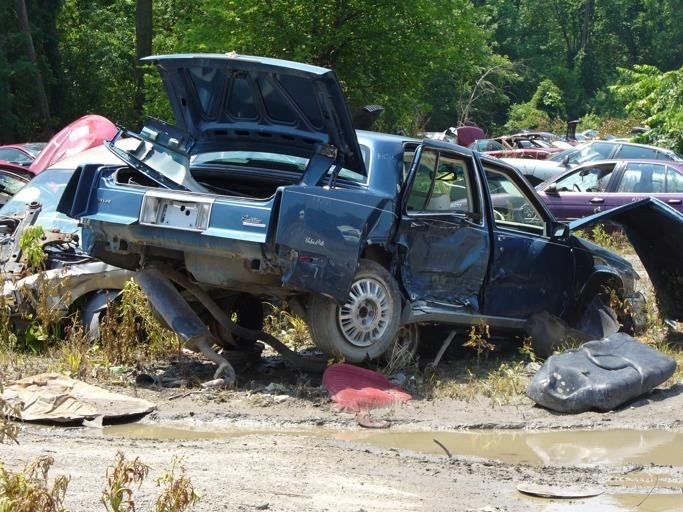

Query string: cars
0 113 130 346
65 52 650 389
402 125 683 226
